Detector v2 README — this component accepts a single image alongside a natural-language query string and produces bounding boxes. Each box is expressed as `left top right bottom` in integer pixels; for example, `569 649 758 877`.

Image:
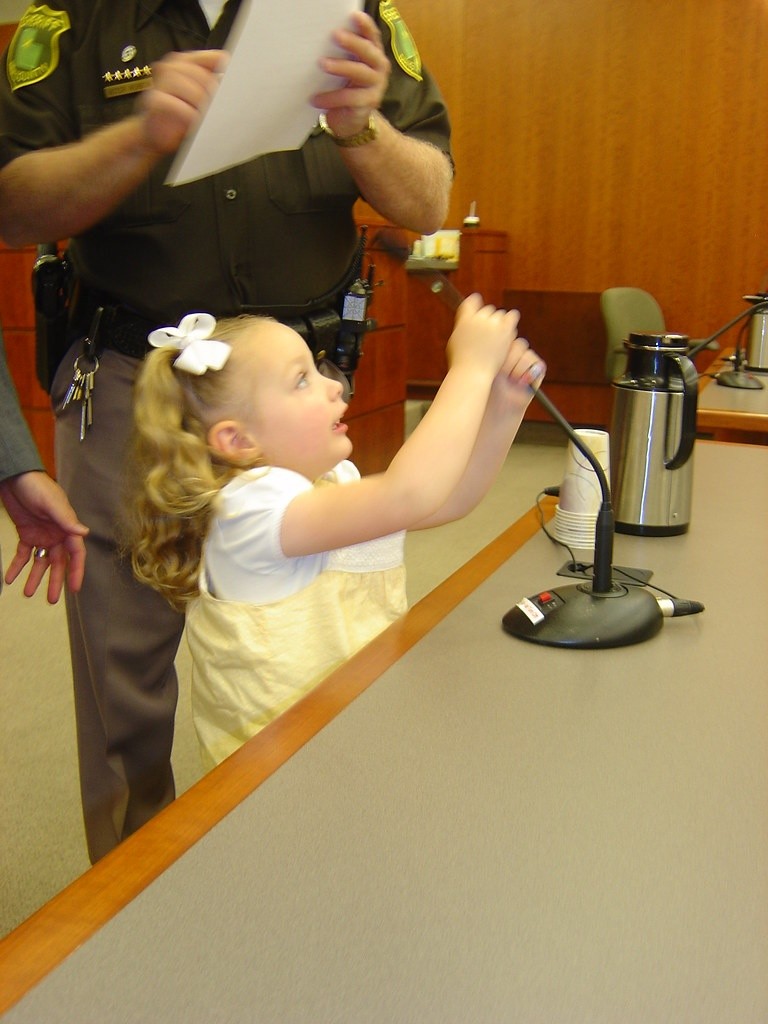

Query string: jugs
744 292 768 372
610 329 699 536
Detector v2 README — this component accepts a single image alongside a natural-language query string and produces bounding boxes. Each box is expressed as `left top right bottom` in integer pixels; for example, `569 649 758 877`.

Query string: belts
76 292 160 359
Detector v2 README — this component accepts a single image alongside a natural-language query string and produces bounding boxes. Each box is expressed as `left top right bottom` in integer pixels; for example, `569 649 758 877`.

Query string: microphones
380 231 666 650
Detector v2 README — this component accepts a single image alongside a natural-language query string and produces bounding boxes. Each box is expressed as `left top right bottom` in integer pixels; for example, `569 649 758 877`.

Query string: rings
35 547 50 558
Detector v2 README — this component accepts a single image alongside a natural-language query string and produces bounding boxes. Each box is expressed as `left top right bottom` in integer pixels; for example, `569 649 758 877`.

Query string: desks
2 440 767 1024
695 344 768 446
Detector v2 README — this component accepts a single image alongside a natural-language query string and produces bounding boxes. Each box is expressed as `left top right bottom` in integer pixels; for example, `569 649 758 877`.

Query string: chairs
599 285 720 385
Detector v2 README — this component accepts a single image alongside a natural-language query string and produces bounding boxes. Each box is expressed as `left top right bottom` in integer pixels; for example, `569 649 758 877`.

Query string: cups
552 429 609 549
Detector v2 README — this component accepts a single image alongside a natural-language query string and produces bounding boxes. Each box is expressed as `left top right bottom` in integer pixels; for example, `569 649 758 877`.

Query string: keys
57 353 99 441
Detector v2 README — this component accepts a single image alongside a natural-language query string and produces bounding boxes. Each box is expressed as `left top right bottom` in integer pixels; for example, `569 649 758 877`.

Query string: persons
124 292 547 804
0 0 456 866
0 324 92 605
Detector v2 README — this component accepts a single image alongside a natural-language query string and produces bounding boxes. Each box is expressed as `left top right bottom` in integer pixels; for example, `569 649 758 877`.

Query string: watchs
316 110 379 149
